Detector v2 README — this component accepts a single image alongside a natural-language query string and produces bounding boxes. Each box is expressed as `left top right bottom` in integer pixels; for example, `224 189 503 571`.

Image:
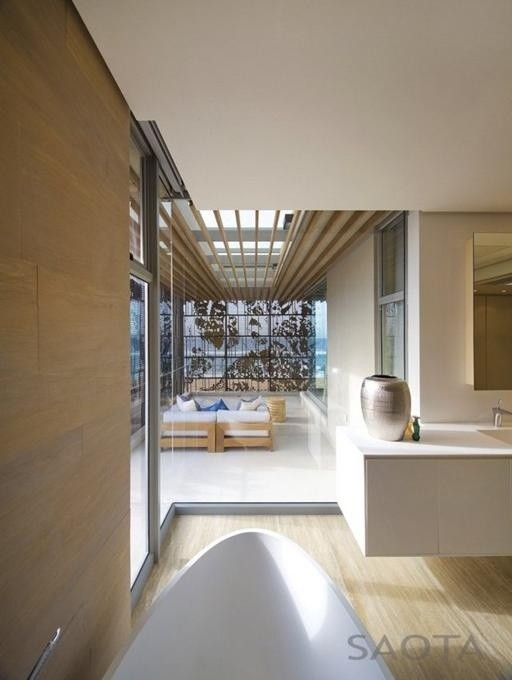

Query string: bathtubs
108 529 393 680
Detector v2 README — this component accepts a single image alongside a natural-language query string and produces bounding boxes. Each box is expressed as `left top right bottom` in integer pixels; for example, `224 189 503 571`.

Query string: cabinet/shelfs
365 457 512 557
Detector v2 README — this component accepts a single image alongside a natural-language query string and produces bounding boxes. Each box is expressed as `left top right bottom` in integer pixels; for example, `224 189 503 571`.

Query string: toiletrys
412 416 421 441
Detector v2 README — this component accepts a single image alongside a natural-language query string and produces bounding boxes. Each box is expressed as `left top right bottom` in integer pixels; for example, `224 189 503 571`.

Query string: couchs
161 391 273 452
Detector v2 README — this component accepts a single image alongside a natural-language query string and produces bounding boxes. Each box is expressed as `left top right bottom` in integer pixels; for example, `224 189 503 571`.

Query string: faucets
491 400 512 427
28 626 61 680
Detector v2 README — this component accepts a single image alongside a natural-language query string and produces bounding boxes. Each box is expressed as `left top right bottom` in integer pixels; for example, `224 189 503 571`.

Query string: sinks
477 429 512 446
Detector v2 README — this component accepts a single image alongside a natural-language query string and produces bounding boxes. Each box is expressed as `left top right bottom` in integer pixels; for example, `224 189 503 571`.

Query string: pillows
176 394 263 413
